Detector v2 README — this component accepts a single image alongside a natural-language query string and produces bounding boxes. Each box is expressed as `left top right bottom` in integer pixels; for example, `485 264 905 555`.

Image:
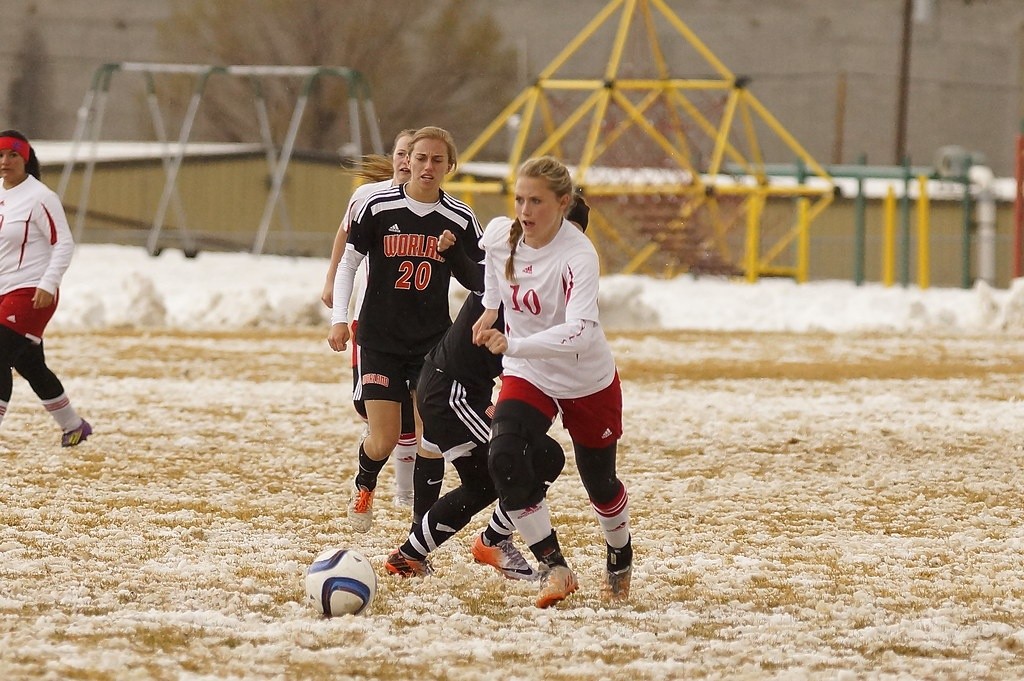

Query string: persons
470 155 632 609
384 192 590 578
320 125 420 511
326 126 485 535
0 129 91 446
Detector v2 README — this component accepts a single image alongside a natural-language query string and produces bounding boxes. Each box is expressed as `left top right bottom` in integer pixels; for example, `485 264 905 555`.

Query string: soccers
303 548 378 619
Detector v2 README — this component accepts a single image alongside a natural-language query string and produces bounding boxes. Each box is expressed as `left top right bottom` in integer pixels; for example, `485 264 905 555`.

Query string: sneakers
61 418 92 448
384 548 434 579
358 425 369 446
348 471 375 533
392 494 411 511
600 562 632 603
536 562 580 608
472 532 536 581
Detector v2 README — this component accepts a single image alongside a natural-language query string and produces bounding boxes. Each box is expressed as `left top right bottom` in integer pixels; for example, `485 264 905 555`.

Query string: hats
565 193 590 232
